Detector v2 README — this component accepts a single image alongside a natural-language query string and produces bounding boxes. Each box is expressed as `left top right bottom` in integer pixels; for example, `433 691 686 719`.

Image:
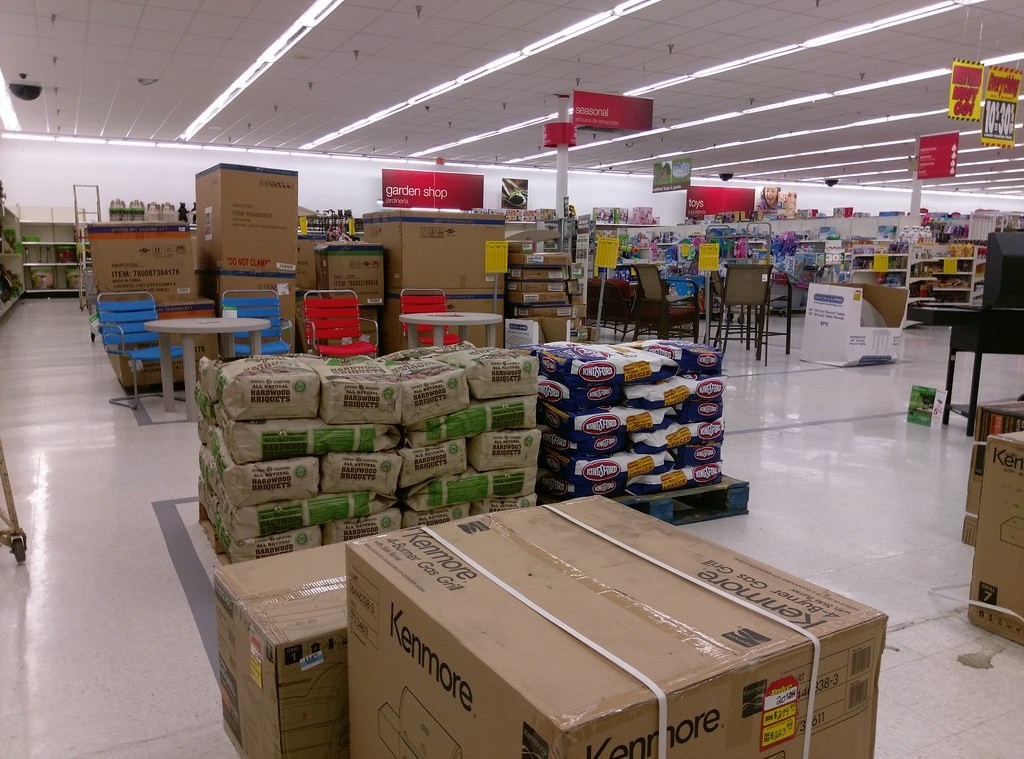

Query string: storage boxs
194 269 297 353
507 282 566 292
967 430 1024 646
296 297 324 359
566 281 579 294
213 526 346 759
569 263 584 280
509 241 533 254
508 304 573 319
313 242 386 307
321 304 380 356
569 294 584 305
509 252 572 266
103 296 220 390
517 317 570 342
506 268 568 282
344 494 890 759
88 221 198 304
572 304 587 319
961 400 1024 547
196 163 298 275
362 211 505 295
508 291 567 305
297 239 320 294
571 320 601 344
380 296 505 355
799 283 910 369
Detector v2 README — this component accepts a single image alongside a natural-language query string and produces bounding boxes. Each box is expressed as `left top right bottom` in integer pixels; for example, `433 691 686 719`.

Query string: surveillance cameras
719 173 734 182
825 180 839 187
8 82 42 102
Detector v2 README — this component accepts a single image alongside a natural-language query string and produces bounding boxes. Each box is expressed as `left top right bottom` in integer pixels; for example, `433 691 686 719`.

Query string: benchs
587 278 636 342
96 292 186 410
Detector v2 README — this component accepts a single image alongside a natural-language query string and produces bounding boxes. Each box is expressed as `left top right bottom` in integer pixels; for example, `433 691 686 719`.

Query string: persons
755 186 783 222
897 226 932 243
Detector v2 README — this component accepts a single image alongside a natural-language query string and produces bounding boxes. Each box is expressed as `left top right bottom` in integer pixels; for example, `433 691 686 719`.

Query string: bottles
110 200 175 222
615 268 630 282
307 209 350 235
299 217 308 234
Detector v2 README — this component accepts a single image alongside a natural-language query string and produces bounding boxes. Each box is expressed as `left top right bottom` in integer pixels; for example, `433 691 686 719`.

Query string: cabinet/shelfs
505 212 1024 326
0 204 92 319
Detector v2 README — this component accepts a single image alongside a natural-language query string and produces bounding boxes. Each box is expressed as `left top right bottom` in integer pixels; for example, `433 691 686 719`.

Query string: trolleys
80 266 98 310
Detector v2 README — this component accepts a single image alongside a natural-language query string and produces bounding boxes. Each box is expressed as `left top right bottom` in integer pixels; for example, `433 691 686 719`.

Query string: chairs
303 290 380 360
712 263 774 367
631 264 699 346
222 289 293 360
400 287 458 347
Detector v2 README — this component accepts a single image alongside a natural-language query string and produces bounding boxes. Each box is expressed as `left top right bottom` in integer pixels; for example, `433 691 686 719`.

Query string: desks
941 305 1024 437
399 312 504 348
143 317 272 423
